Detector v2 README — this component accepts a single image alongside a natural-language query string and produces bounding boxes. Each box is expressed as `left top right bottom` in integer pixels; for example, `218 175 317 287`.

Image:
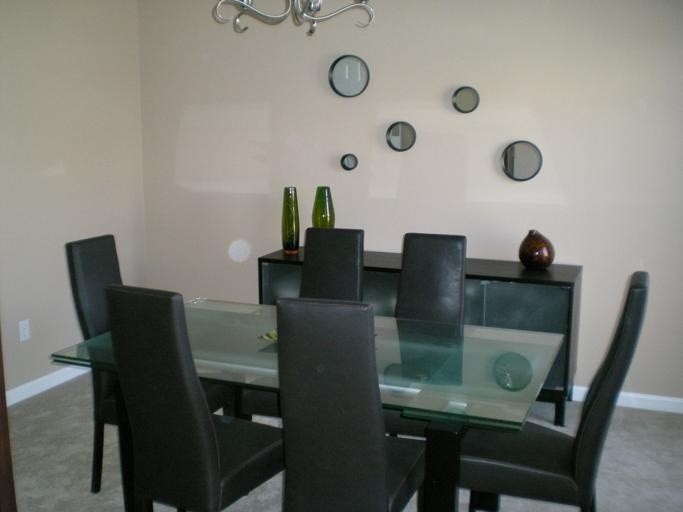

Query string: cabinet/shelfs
258 250 585 425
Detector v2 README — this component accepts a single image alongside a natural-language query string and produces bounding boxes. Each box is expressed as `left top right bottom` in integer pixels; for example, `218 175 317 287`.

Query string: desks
48 296 566 512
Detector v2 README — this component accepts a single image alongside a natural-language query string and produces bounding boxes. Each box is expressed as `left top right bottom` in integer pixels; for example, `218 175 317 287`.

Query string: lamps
212 0 375 36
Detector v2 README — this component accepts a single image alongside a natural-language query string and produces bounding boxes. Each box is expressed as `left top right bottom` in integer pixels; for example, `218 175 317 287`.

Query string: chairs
66 233 235 493
238 228 364 424
383 232 465 437
103 280 284 512
456 271 648 512
275 298 427 510
395 318 465 387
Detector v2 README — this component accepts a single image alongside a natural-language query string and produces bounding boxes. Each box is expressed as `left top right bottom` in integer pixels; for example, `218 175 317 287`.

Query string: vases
311 186 335 228
282 186 298 255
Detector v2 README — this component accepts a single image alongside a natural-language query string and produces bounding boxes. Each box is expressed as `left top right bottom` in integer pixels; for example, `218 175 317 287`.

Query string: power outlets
19 318 31 342
